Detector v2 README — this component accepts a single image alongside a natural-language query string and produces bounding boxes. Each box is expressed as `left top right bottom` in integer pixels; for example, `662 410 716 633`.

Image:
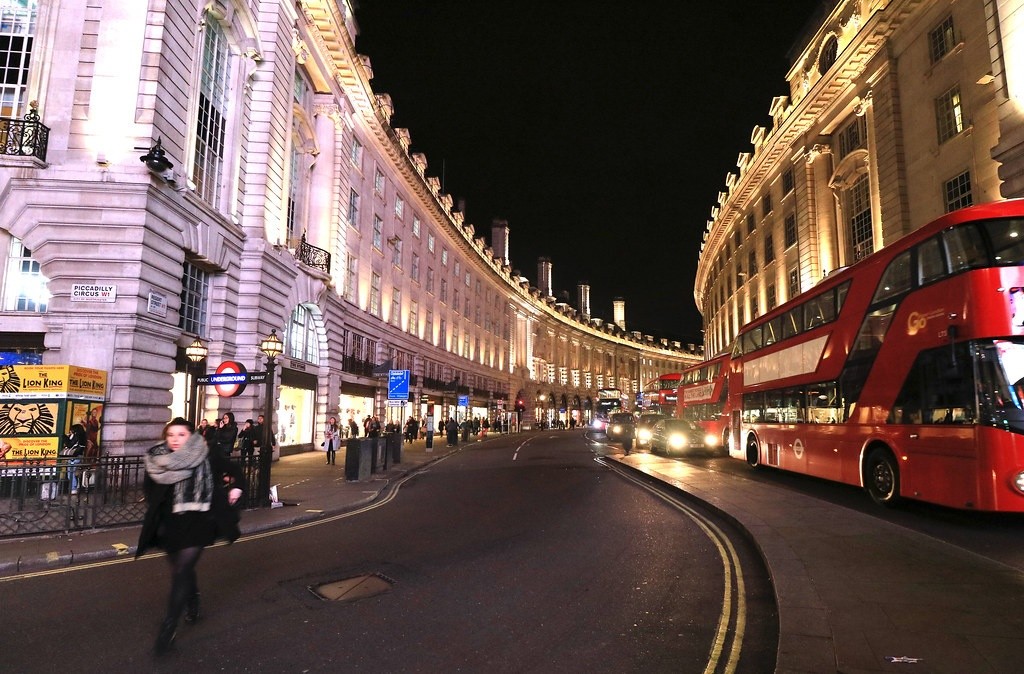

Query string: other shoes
183 608 198 624
332 462 335 464
153 617 176 653
326 461 329 465
70 490 78 495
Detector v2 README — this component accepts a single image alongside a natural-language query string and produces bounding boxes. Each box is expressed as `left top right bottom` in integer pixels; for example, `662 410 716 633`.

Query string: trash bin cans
372 432 405 475
345 437 372 482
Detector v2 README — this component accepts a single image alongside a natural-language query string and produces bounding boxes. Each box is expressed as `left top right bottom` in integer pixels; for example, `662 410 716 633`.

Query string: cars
649 419 719 458
604 413 637 441
635 415 668 447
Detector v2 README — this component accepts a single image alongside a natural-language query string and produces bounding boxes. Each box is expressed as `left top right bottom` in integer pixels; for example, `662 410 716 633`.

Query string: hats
245 419 254 426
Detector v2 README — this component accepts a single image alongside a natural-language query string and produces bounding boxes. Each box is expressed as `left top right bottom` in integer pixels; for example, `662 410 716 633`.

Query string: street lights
185 337 209 433
257 329 284 505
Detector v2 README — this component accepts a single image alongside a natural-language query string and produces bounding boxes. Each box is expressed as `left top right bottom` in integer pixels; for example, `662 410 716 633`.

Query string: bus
677 355 733 452
725 198 1024 513
643 373 683 416
595 388 627 433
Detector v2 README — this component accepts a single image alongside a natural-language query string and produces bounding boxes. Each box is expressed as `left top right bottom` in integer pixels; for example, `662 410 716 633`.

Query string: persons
0 443 11 459
135 416 245 654
582 420 585 428
438 417 508 445
324 417 340 465
551 418 575 430
623 429 632 452
364 415 381 438
541 419 545 431
62 424 86 495
386 420 401 433
196 412 276 480
403 416 427 444
349 419 359 438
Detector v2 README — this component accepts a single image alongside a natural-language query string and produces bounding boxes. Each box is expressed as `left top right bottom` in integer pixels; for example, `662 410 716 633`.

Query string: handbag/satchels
58 443 80 457
232 438 243 452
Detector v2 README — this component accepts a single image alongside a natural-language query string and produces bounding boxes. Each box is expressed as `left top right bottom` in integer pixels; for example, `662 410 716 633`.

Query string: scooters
622 428 635 453
